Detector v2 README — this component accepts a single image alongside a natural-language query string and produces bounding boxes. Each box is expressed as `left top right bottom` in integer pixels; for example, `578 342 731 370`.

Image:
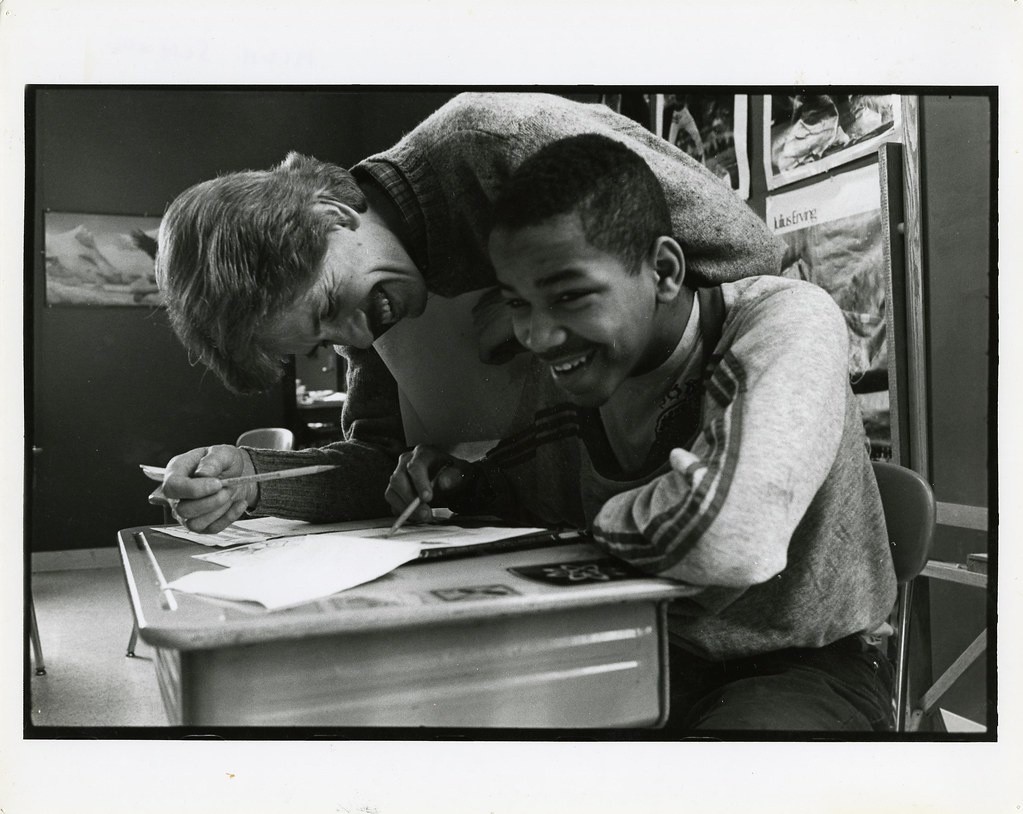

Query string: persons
156 92 789 533
382 133 901 729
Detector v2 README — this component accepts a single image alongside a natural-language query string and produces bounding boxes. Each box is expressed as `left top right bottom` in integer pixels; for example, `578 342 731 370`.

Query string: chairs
871 459 935 733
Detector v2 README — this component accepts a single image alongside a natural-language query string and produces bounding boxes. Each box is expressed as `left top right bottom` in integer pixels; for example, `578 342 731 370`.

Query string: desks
117 513 703 729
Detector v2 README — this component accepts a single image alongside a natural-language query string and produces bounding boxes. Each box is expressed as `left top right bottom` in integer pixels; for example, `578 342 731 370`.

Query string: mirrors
286 343 347 419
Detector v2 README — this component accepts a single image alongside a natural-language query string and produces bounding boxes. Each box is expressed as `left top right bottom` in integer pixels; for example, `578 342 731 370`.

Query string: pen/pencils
221 465 341 487
386 482 440 538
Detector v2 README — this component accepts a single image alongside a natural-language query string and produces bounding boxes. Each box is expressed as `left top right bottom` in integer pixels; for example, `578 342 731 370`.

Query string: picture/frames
42 207 167 308
